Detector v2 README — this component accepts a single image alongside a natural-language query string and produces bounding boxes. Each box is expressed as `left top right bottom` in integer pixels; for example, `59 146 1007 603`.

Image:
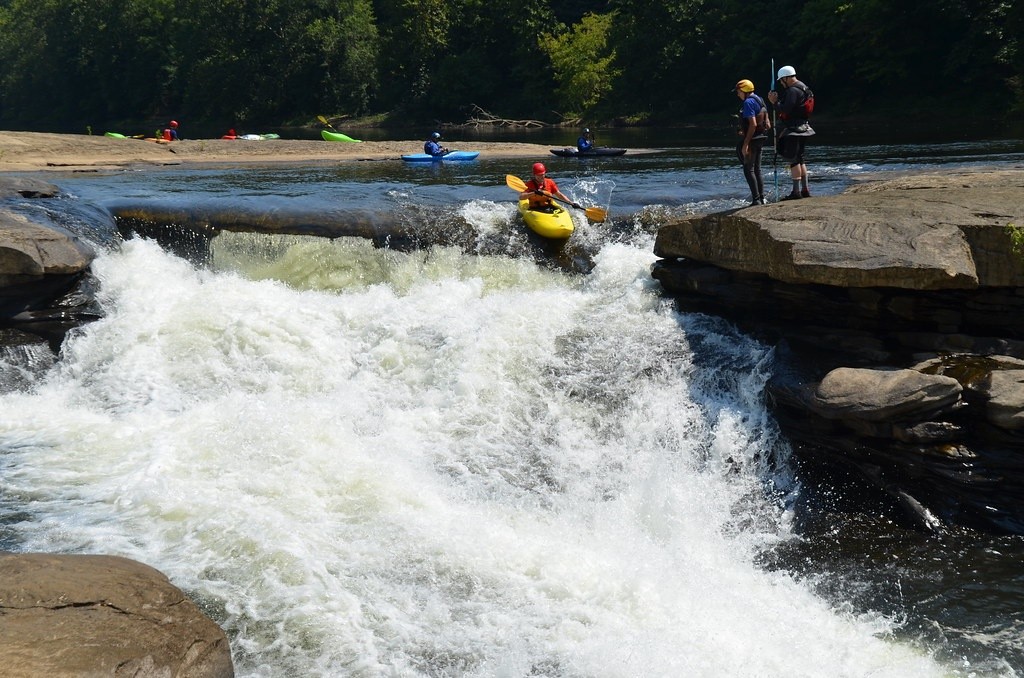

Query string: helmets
533 163 546 175
433 132 441 139
583 128 590 133
736 79 755 93
776 65 796 80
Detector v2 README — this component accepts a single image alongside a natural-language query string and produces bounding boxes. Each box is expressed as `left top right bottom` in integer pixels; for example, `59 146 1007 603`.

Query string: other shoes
749 199 766 206
786 192 810 198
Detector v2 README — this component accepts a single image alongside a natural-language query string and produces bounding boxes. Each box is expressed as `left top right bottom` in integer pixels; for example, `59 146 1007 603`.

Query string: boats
105 132 145 139
321 130 362 143
401 150 479 161
549 147 627 157
223 133 279 140
519 195 575 240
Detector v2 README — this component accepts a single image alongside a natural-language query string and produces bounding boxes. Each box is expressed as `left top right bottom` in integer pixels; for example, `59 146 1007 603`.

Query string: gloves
535 190 543 196
572 203 580 208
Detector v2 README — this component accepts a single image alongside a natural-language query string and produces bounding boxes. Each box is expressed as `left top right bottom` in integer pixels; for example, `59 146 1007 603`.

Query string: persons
578 128 593 153
731 79 771 207
519 163 580 213
169 120 180 141
429 132 449 156
768 66 815 199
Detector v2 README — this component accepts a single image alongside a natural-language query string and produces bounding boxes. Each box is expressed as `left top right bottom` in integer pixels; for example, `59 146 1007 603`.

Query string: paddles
588 123 596 151
316 114 338 134
505 173 609 221
